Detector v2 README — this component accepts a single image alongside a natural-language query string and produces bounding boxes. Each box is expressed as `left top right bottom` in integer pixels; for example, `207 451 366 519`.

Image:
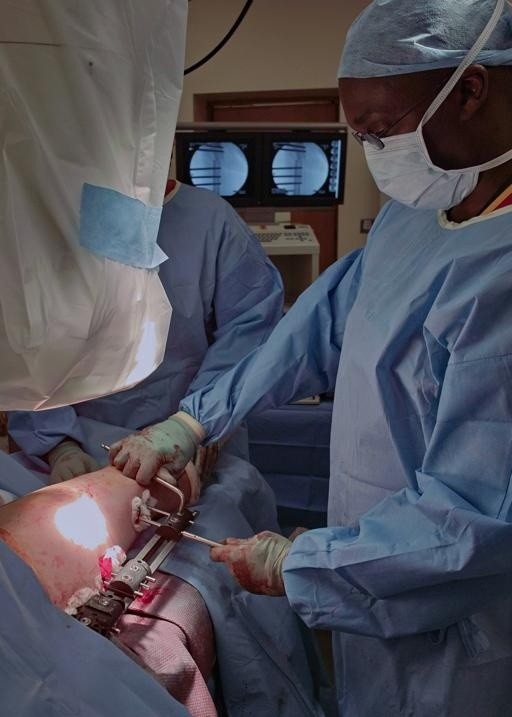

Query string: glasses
350 72 482 152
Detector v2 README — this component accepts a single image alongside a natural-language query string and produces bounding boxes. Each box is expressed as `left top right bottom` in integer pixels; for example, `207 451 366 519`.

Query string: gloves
109 416 203 487
46 441 100 486
209 530 293 597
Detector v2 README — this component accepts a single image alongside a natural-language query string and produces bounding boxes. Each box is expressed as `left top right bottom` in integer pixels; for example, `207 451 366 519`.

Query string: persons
107 1 512 717
7 178 284 483
0 441 192 613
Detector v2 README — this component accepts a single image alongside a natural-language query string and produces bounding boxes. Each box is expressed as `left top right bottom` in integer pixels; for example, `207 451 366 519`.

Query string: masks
363 133 479 212
